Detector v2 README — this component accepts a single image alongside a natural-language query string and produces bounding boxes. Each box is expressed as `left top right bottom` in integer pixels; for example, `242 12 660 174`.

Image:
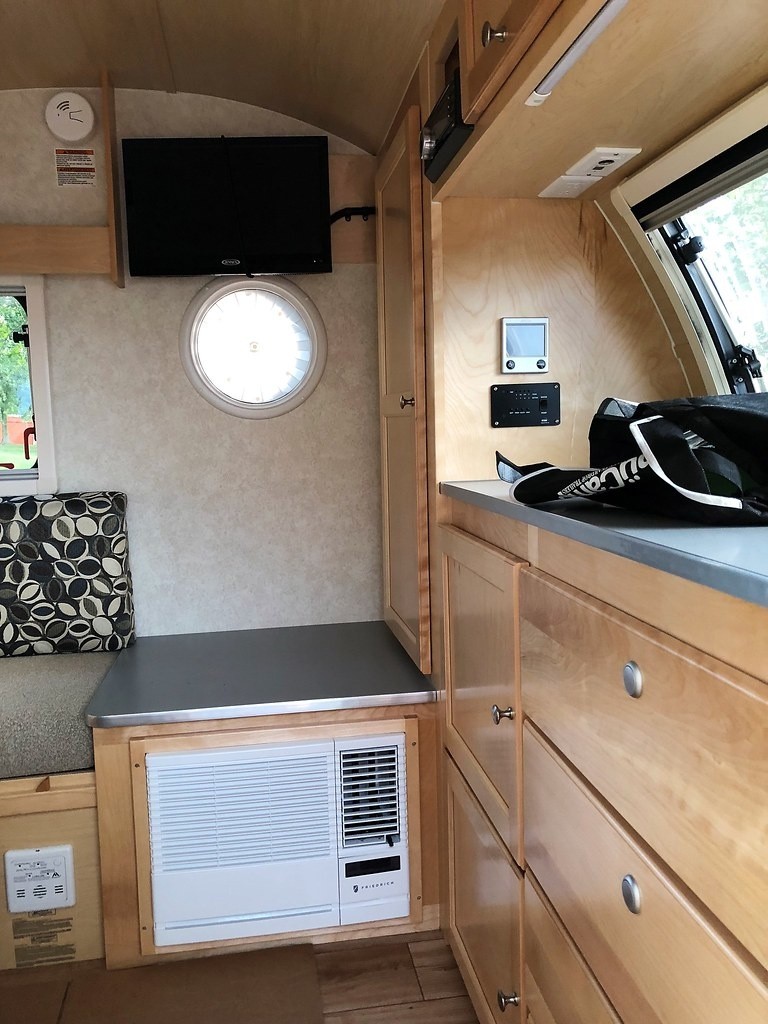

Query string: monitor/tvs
122 135 333 276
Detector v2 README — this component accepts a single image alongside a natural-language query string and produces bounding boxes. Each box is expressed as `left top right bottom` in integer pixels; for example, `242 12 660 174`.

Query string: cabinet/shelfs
372 0 768 1024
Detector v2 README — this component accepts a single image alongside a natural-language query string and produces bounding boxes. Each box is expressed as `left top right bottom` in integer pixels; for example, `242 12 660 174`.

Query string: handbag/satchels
511 392 767 523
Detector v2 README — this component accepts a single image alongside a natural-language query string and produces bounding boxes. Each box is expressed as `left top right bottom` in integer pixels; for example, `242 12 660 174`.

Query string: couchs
0 491 135 1024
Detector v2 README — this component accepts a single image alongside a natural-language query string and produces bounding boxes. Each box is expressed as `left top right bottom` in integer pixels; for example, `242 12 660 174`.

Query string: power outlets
537 176 603 198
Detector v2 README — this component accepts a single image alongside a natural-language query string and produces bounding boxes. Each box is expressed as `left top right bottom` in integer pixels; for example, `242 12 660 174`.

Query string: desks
84 621 423 972
